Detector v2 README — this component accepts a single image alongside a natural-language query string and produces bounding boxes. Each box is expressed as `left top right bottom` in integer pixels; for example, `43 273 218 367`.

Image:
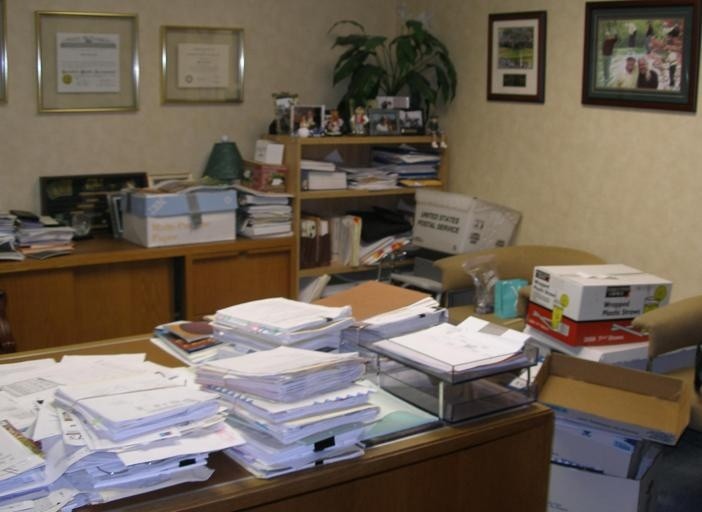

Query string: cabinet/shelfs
0 257 174 353
186 246 291 319
262 135 448 301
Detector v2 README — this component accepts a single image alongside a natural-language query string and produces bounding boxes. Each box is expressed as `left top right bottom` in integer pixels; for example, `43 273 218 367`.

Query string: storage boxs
530 262 673 322
412 187 521 256
532 355 693 443
525 303 649 346
523 325 651 371
552 420 644 479
123 186 238 247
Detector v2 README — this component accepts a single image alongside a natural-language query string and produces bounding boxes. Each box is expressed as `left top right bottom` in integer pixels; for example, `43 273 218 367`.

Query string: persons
597 19 678 92
351 106 369 135
376 116 389 132
425 114 448 149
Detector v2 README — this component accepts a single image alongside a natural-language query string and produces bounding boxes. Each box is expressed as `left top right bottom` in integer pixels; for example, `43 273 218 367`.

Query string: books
300 158 347 190
1 214 74 262
302 215 411 269
311 281 450 347
234 183 293 241
153 319 241 366
194 345 380 479
338 164 398 191
211 296 354 351
155 174 232 197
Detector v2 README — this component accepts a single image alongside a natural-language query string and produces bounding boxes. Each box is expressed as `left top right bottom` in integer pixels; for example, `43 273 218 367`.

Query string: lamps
203 135 245 186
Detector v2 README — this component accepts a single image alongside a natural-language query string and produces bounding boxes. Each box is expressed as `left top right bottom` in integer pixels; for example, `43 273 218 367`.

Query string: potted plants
323 19 459 134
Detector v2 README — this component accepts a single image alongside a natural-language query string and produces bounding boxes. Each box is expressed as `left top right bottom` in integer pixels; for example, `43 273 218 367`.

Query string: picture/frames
581 0 701 113
487 11 547 105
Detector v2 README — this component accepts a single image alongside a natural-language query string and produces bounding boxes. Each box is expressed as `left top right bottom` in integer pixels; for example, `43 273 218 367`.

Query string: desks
0 305 556 512
